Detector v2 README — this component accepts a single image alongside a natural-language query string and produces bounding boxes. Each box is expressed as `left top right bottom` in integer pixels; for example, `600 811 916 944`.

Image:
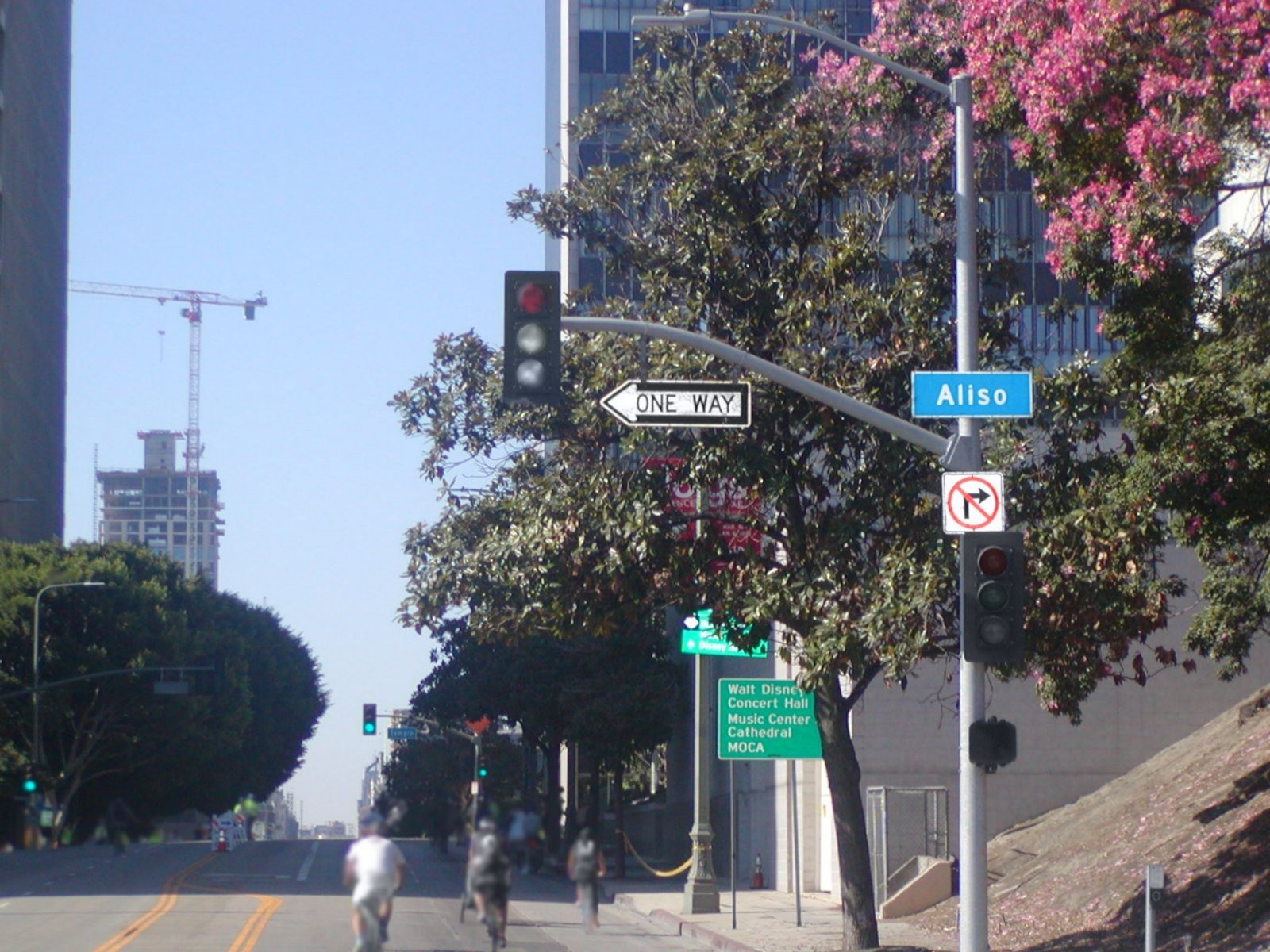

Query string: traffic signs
600 378 754 426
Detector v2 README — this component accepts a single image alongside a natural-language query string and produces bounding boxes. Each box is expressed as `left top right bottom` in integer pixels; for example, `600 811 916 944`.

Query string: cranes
68 279 268 580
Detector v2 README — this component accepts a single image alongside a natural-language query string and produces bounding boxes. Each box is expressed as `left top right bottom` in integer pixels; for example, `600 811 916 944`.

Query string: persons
507 804 544 873
343 813 407 952
568 829 606 926
467 818 510 948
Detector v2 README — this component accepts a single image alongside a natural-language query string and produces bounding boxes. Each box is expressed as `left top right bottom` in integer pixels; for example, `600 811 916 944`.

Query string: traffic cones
215 829 228 852
750 853 769 890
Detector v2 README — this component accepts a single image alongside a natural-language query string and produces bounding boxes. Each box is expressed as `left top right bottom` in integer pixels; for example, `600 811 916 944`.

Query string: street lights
32 581 106 851
631 6 989 952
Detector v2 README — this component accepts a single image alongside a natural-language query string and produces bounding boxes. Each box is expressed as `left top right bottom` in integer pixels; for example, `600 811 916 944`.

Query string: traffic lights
959 531 1027 664
20 780 41 796
479 755 488 777
363 704 376 735
505 270 562 401
969 718 1019 763
